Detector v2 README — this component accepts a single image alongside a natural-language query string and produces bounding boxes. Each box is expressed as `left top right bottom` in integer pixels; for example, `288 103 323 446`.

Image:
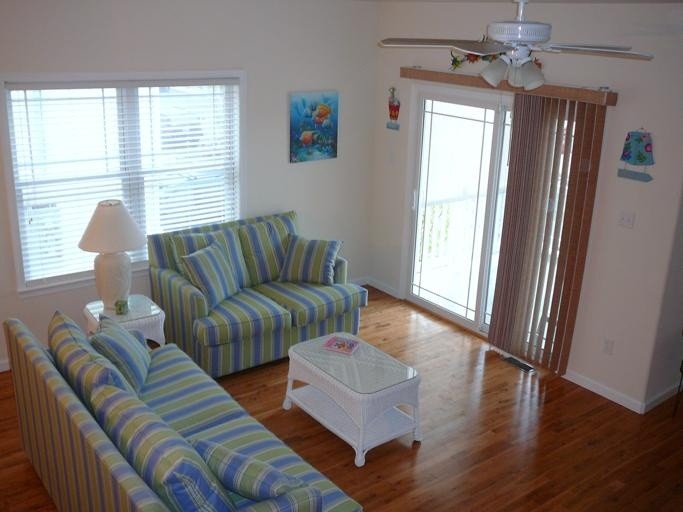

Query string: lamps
78 199 148 310
479 46 546 91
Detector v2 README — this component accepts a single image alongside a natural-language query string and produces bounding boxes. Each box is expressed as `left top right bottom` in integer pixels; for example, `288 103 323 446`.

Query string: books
320 335 360 355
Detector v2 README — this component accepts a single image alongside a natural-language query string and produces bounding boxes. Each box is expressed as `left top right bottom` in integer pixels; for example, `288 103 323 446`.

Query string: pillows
186 437 308 500
169 228 252 289
91 383 240 512
48 309 139 416
239 210 298 286
182 241 242 313
89 312 152 396
278 233 344 288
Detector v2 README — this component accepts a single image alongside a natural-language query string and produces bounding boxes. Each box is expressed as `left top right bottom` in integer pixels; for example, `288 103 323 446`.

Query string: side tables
83 294 166 351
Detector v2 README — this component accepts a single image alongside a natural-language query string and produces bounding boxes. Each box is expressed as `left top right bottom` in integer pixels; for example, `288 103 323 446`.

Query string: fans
380 0 655 62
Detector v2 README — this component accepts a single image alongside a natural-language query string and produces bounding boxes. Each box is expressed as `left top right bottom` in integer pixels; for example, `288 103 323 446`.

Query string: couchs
147 210 368 379
4 317 364 512
